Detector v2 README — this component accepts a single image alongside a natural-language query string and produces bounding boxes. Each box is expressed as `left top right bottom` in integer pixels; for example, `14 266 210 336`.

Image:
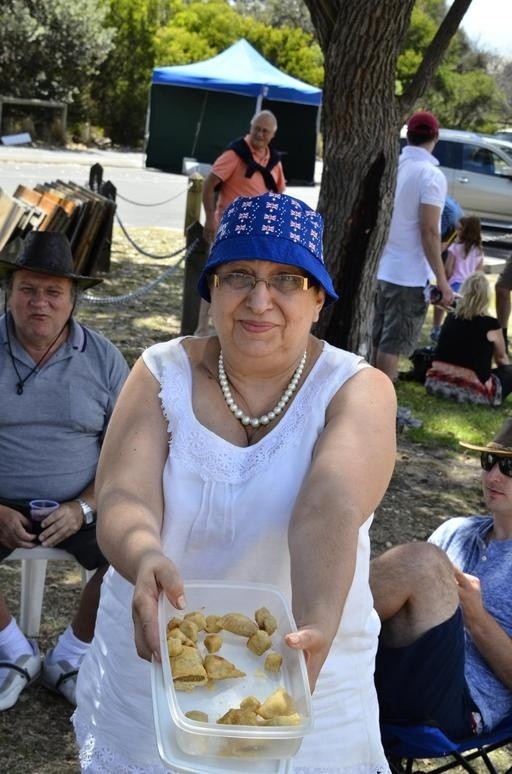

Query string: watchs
72 497 95 529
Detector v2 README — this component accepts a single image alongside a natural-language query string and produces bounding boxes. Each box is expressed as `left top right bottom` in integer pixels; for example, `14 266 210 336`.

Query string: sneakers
0 639 42 710
44 646 85 704
397 407 423 428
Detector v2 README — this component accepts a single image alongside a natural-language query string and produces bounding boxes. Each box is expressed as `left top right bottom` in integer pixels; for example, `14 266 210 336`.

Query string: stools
1 544 89 643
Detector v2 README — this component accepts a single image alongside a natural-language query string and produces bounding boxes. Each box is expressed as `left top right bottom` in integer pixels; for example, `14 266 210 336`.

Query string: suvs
399 124 512 238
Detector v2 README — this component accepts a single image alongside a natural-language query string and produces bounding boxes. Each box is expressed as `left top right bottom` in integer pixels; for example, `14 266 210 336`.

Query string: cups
28 499 61 544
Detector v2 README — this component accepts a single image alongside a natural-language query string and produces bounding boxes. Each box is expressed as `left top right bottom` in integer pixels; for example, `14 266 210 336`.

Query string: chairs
383 718 511 773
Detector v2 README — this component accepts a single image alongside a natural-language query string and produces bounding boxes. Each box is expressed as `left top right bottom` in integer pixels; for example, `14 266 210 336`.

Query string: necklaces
215 346 309 429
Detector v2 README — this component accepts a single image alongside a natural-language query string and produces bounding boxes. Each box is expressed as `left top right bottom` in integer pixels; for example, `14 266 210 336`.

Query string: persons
430 192 470 343
60 187 397 774
0 229 133 712
365 106 467 435
495 244 512 359
358 415 511 757
421 272 512 410
444 215 488 322
201 110 291 247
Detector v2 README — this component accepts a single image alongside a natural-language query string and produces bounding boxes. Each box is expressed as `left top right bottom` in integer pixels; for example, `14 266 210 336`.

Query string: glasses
480 452 512 475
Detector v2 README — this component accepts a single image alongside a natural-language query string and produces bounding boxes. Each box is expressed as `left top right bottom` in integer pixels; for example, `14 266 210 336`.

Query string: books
0 177 118 270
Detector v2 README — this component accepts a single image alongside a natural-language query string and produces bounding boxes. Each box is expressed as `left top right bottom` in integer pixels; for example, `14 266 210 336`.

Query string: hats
0 231 103 289
407 111 440 133
458 418 512 453
197 192 340 309
